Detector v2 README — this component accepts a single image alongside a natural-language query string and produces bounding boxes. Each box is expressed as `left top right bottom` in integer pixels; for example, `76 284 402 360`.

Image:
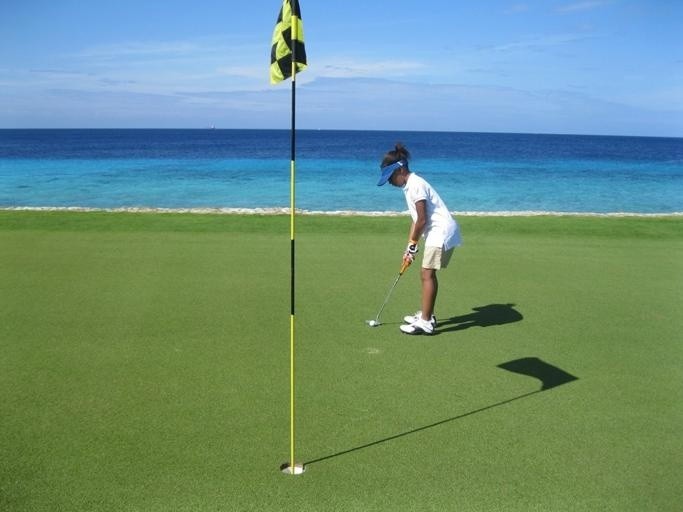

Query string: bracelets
408 239 418 244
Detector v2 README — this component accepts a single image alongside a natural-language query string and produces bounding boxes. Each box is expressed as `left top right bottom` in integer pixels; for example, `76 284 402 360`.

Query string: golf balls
369 320 375 326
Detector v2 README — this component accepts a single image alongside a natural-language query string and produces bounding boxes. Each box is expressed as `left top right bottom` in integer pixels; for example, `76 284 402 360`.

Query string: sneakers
404 312 437 327
399 320 434 336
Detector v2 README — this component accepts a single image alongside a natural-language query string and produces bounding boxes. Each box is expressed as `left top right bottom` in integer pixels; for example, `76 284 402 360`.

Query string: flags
270 0 307 85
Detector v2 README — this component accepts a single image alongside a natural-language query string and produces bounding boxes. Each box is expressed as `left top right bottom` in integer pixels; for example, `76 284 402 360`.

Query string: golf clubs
366 254 412 325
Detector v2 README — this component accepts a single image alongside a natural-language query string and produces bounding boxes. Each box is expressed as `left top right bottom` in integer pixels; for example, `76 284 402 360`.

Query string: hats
377 159 407 186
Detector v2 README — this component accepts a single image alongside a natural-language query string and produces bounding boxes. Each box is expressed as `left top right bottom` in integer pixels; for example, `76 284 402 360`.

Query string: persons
376 143 462 335
